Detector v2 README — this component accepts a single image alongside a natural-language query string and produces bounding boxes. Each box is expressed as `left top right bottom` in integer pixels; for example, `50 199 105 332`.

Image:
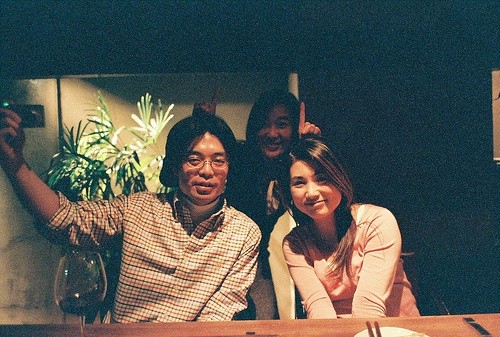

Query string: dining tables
0 313 500 337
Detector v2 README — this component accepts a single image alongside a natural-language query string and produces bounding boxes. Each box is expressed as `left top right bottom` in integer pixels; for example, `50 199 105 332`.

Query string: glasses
185 156 230 169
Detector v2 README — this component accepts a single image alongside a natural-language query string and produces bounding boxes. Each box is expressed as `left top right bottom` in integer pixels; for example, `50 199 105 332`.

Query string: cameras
4 104 45 128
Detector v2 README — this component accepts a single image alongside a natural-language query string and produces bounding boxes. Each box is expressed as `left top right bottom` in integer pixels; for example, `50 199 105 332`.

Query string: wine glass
55 252 107 337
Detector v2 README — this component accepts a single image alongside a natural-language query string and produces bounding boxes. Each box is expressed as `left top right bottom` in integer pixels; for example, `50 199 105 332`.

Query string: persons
276 134 421 319
0 109 263 322
192 90 323 322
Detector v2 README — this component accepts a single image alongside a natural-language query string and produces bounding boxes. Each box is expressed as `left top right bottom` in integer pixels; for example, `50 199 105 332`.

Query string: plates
354 327 429 337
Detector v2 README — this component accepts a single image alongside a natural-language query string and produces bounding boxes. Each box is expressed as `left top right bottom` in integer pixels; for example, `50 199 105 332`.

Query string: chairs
269 210 300 320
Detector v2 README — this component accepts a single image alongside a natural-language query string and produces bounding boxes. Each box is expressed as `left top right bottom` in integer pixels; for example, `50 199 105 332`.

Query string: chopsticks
366 321 382 337
